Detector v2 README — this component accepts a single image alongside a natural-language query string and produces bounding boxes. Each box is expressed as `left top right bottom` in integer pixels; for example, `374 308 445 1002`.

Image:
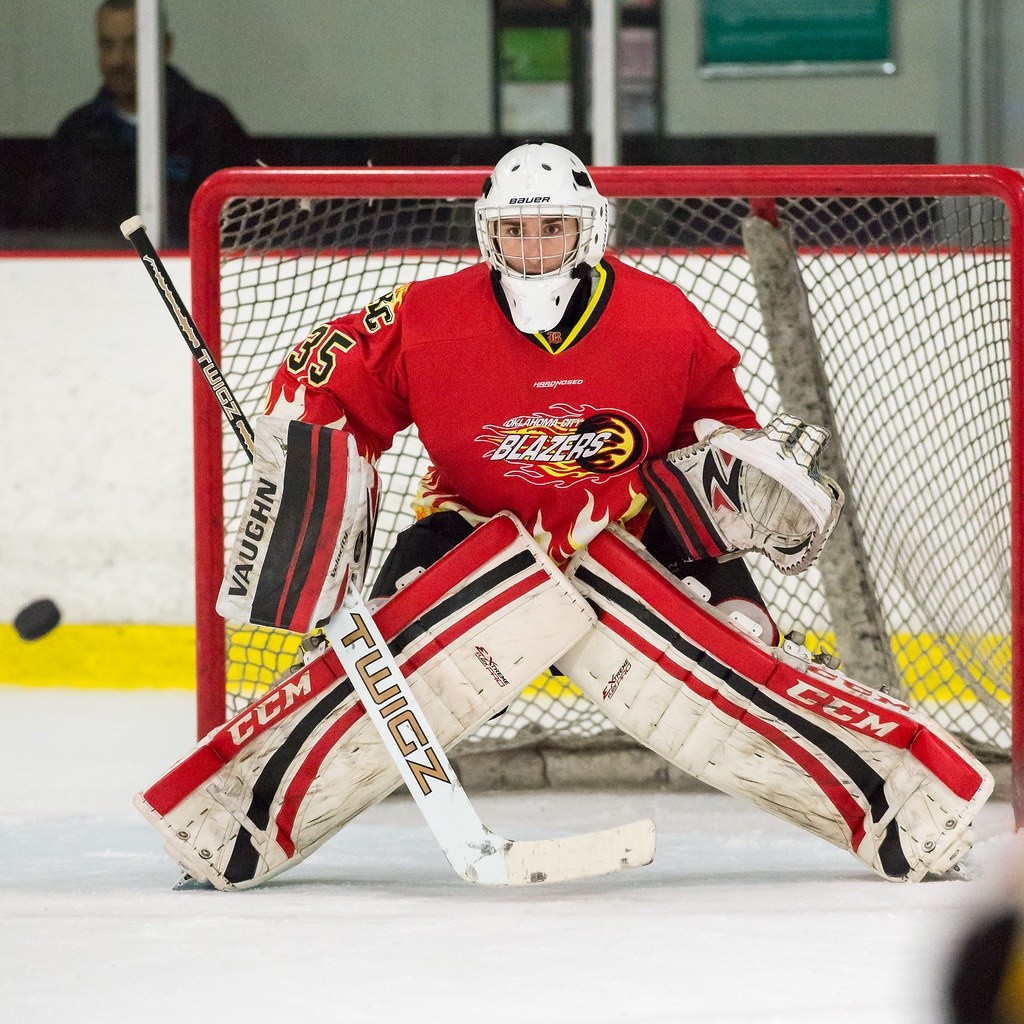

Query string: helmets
474 143 609 334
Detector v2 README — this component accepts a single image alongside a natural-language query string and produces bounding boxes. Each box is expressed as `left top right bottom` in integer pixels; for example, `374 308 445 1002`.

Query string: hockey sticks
118 213 656 888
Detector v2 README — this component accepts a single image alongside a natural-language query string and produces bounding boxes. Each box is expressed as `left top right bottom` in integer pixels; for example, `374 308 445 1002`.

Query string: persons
133 142 995 891
35 0 264 248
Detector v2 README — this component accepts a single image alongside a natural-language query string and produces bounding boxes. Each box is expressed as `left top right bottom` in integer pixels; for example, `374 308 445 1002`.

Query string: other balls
13 599 62 642
